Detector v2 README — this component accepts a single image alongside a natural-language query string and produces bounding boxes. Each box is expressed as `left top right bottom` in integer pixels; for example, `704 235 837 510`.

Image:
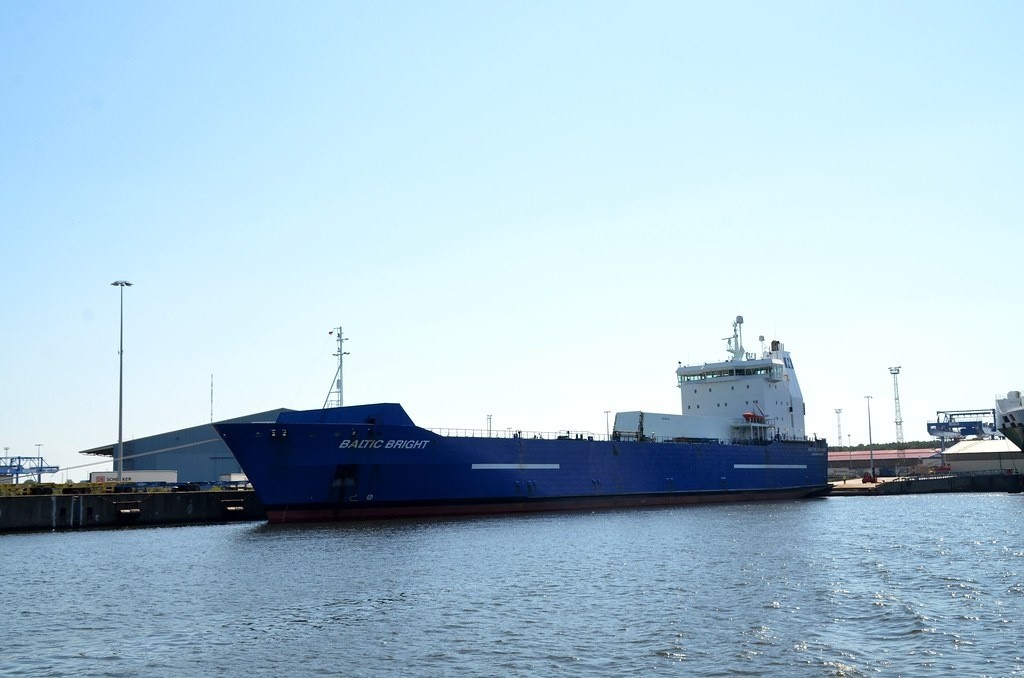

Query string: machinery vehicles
863 467 877 483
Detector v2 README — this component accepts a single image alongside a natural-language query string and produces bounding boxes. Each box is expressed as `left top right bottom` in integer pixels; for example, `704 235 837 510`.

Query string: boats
211 315 836 523
996 391 1024 454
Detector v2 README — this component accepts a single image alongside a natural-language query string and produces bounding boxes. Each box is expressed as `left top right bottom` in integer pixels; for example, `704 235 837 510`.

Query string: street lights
848 434 853 470
111 280 133 479
603 411 612 440
36 444 43 481
507 428 513 437
865 395 874 477
4 447 9 464
487 415 493 437
